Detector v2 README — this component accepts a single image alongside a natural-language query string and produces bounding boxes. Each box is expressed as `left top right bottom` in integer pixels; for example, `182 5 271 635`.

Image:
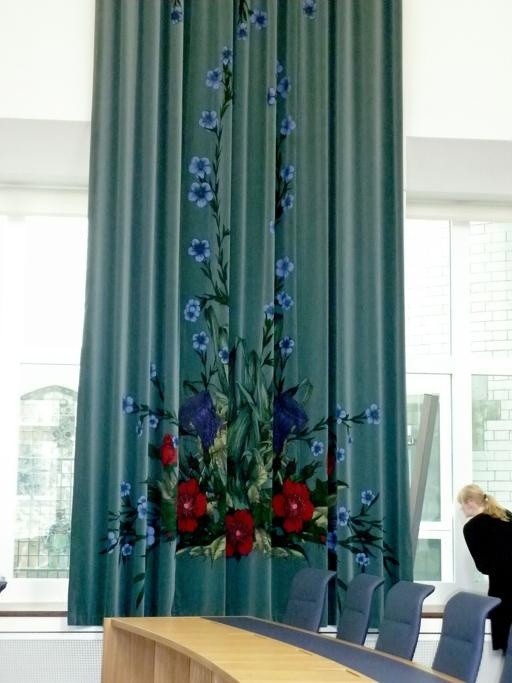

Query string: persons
458 484 512 651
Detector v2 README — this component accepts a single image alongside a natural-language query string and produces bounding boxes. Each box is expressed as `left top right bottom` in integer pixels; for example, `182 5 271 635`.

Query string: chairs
281 567 335 632
374 581 434 661
334 572 385 647
431 591 502 683
496 625 512 683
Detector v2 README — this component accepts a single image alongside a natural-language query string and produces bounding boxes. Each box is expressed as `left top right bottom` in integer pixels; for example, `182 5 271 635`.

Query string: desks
102 617 467 683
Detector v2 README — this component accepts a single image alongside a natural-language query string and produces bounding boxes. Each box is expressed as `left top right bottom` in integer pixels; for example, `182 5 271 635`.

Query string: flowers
98 0 400 617
